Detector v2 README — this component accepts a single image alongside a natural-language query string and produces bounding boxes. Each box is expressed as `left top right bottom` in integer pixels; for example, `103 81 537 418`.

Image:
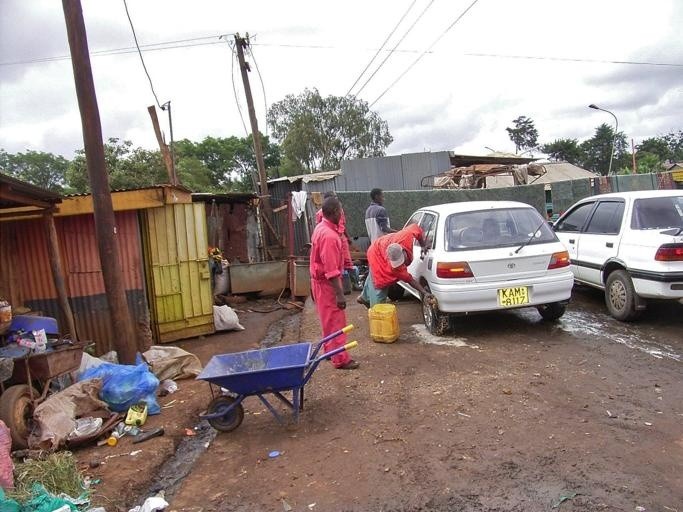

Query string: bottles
16 337 36 350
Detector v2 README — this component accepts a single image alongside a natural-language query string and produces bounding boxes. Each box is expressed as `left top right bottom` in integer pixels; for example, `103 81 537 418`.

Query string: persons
356 222 432 310
309 195 361 369
315 191 364 292
364 187 399 245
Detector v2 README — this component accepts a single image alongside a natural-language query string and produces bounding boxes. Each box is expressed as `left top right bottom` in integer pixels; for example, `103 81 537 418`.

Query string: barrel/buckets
125 404 148 427
0 304 12 335
368 303 399 343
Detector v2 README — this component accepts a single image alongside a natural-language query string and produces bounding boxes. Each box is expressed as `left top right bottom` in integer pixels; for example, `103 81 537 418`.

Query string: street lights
589 104 619 178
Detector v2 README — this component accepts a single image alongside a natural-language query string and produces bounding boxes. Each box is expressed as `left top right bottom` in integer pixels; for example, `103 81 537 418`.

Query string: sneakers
356 296 370 308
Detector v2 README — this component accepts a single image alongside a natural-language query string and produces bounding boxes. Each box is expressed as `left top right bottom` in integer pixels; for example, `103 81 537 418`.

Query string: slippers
337 360 360 369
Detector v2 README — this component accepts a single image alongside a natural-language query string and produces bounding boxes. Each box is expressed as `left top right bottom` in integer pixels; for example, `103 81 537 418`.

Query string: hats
386 243 405 268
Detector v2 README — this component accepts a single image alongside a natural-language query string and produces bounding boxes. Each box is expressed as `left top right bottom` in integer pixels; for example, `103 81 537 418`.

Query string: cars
403 198 575 336
552 188 683 322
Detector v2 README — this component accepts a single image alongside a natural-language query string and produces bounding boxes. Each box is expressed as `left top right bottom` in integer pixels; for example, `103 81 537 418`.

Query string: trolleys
0 341 97 450
193 324 358 433
